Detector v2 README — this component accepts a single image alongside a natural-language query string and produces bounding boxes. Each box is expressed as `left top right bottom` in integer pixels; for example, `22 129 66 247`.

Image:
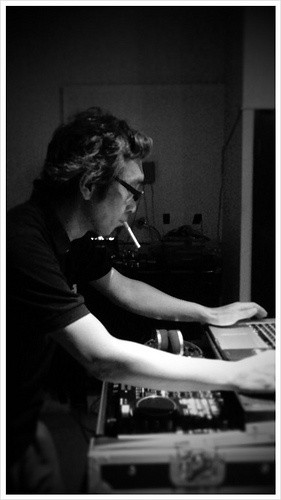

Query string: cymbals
169 226 208 242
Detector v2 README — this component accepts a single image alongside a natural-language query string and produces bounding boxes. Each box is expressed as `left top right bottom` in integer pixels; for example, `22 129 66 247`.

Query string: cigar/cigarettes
124 221 141 249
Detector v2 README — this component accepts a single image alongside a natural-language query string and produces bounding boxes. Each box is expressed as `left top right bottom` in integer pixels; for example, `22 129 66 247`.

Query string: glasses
106 174 144 200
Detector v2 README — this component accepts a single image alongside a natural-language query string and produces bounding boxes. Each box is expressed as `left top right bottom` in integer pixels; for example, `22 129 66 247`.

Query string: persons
6 106 275 479
